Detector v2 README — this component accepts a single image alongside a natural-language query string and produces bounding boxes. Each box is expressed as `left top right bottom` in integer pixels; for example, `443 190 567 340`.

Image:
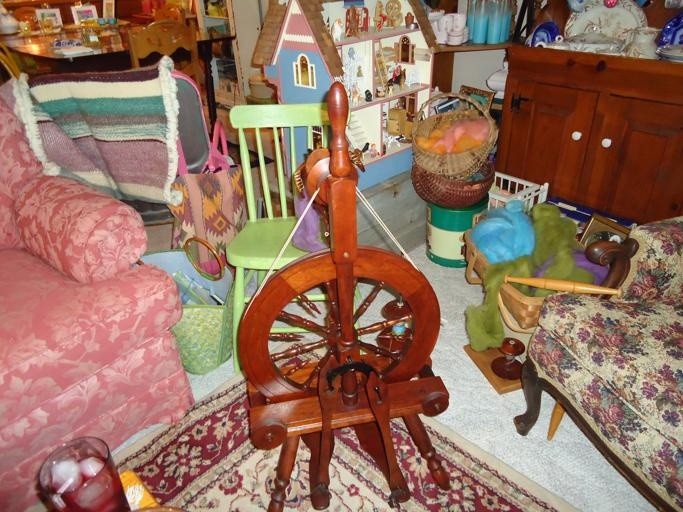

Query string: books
216 58 235 72
202 16 227 33
54 46 93 56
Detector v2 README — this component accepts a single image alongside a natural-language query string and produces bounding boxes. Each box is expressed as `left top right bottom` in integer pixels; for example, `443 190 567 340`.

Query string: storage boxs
462 230 596 333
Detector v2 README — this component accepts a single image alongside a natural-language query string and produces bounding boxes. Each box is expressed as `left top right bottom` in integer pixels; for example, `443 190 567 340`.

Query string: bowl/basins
525 21 560 47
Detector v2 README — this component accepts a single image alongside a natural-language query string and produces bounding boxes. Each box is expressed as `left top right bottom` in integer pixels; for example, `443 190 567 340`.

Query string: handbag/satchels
165 119 250 276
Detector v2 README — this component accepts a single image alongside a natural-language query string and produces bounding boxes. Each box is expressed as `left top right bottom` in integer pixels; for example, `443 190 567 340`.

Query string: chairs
24 68 231 250
130 16 200 90
225 104 360 374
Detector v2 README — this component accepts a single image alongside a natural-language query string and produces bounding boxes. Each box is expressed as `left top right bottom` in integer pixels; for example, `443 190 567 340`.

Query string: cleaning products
467 0 512 44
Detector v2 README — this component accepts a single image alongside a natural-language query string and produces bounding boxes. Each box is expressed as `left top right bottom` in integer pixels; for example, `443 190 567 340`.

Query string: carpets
110 342 589 511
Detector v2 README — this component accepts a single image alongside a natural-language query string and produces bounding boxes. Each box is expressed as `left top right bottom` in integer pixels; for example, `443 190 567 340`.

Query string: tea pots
625 27 662 59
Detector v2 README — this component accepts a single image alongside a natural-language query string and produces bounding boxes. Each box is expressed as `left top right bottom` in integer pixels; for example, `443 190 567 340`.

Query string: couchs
0 97 194 512
514 217 683 512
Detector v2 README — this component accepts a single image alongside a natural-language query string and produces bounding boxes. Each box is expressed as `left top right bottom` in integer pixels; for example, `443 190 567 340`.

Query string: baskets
464 228 548 334
410 160 496 208
411 93 499 178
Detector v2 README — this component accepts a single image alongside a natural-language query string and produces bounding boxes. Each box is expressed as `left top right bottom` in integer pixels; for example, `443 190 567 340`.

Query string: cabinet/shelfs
495 44 683 226
251 0 437 199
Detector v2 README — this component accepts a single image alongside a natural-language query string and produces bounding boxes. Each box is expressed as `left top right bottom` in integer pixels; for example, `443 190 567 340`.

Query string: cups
37 437 131 512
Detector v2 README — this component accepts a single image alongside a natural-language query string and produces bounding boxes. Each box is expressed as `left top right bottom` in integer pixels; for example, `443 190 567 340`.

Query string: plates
658 12 683 45
656 44 683 63
564 0 647 40
